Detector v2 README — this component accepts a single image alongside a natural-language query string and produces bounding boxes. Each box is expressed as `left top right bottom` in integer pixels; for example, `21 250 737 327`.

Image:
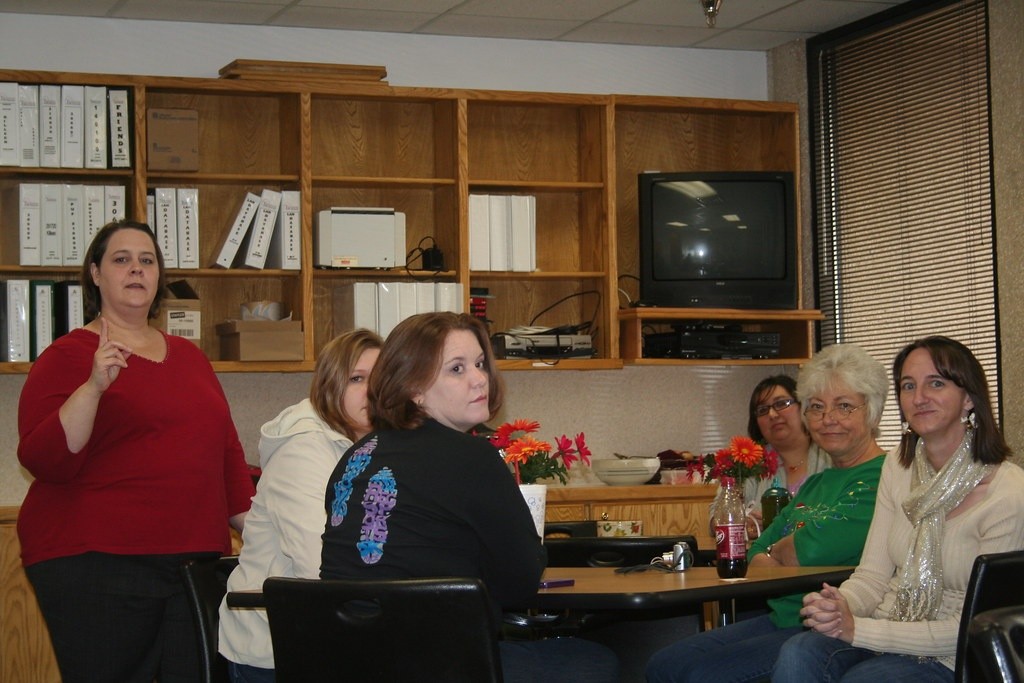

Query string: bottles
714 476 747 578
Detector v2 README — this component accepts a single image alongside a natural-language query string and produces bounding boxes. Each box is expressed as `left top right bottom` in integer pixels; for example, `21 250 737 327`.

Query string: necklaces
778 449 809 476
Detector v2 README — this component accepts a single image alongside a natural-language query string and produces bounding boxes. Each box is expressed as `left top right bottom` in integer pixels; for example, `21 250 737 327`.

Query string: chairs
260 576 506 683
178 553 229 683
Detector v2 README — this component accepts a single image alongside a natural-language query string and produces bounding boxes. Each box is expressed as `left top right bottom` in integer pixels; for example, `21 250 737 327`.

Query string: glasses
803 402 866 421
753 399 797 416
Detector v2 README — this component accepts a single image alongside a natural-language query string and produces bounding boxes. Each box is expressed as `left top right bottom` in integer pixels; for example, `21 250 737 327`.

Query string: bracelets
766 543 775 558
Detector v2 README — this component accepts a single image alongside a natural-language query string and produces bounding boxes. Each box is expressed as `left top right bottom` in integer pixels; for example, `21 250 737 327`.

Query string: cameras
661 541 690 572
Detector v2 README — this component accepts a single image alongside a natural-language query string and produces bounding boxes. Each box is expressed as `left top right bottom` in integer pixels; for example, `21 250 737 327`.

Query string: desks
534 559 858 635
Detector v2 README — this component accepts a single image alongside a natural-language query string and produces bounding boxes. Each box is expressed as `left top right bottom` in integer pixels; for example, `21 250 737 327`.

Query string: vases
520 484 546 541
705 481 724 535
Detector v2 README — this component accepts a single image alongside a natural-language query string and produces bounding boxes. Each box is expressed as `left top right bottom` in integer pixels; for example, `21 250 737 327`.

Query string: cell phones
538 579 576 588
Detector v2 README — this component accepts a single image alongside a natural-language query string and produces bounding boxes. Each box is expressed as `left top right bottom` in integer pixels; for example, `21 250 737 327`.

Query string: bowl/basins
591 458 661 486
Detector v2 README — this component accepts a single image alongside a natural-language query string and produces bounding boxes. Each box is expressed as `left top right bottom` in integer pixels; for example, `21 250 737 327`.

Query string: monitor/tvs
637 170 799 311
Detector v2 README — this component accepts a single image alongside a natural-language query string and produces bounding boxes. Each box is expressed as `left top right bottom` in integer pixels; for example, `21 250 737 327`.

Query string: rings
747 527 754 534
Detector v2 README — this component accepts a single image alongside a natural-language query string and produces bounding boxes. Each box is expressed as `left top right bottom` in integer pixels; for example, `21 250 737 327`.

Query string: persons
708 373 836 627
643 343 890 683
14 218 258 683
769 335 1024 683
217 326 386 683
317 310 624 683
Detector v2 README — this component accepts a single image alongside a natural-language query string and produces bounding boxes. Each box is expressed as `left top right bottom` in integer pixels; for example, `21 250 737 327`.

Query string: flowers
687 434 777 492
484 419 592 485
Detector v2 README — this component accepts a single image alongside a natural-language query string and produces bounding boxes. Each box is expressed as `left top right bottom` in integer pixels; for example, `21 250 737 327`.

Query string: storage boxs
210 320 306 362
330 278 466 342
149 298 203 348
0 82 203 270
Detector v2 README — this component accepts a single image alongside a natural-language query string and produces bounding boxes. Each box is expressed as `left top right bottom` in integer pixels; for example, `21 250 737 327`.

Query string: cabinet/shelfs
0 69 817 372
537 499 720 554
